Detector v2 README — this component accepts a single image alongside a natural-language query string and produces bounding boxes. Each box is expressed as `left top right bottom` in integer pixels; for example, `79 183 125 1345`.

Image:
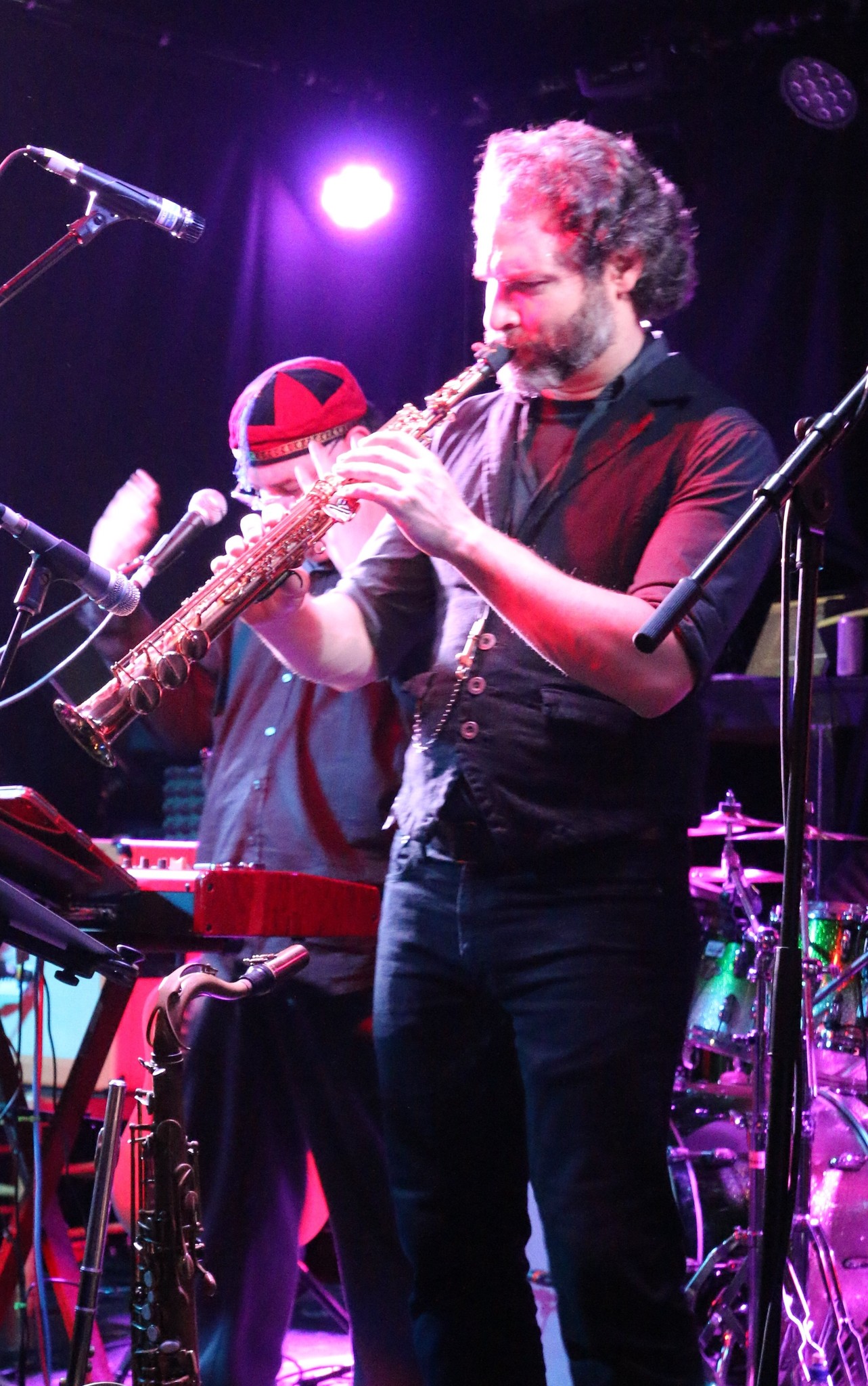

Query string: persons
210 118 781 1385
81 356 424 1386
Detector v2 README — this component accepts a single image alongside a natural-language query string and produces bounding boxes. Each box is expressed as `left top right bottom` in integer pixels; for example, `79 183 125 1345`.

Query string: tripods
681 825 867 1385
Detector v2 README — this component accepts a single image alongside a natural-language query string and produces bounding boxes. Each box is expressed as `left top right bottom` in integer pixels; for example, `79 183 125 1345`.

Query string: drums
684 1091 868 1386
684 942 765 1065
798 901 868 1048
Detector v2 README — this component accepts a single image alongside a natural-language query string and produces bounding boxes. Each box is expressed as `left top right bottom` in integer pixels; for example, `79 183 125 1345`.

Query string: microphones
25 145 206 243
130 489 228 588
0 504 140 616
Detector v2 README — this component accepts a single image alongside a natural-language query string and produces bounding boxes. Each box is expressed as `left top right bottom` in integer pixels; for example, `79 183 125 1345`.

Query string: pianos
2 784 248 1386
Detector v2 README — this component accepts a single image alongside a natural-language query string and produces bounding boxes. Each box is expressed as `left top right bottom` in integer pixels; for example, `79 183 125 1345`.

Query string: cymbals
671 1080 758 1098
688 866 784 883
701 811 784 828
725 823 868 841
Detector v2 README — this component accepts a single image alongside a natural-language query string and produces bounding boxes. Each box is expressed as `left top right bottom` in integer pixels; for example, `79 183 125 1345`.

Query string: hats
229 357 372 466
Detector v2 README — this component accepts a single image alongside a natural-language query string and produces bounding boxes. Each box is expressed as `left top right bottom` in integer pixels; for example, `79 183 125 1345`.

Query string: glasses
232 479 302 512
